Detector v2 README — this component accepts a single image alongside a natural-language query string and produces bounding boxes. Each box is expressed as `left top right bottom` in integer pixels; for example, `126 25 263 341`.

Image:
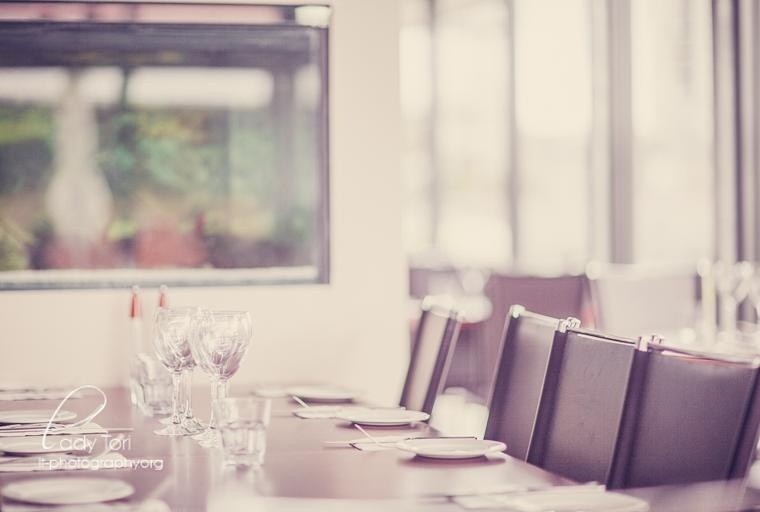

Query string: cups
209 396 273 472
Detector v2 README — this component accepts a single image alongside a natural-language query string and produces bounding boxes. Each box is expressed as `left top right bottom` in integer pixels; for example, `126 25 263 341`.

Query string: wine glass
152 306 250 446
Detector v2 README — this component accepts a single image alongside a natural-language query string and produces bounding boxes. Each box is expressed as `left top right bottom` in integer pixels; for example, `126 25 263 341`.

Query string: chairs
382 255 755 510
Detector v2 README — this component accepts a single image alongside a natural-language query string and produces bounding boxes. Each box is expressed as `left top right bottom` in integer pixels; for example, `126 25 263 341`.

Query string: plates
283 390 508 460
1 408 134 503
